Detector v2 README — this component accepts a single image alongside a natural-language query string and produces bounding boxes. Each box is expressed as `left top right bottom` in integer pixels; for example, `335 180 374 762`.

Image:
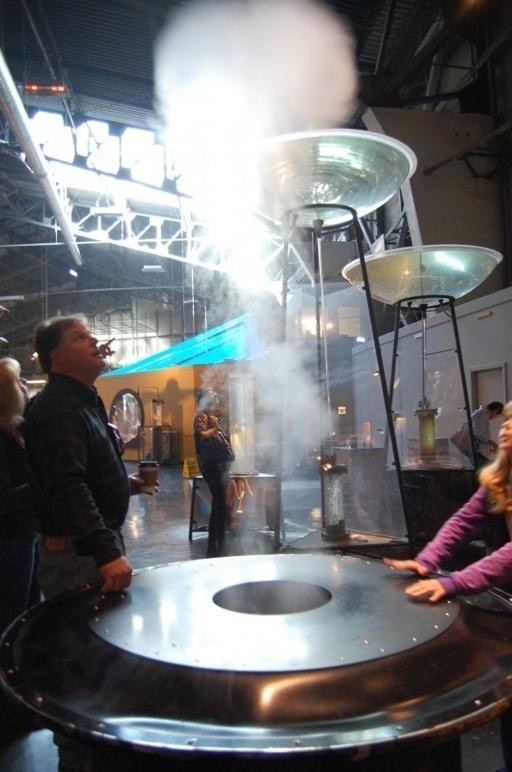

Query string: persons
383 400 512 772
23 312 159 772
466 401 504 465
0 357 46 732
194 388 231 557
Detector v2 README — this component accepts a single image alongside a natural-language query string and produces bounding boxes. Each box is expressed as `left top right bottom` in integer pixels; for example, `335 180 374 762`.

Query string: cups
137 460 160 492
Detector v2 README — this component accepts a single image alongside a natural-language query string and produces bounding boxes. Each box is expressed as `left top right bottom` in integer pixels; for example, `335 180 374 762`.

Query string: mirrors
108 388 145 448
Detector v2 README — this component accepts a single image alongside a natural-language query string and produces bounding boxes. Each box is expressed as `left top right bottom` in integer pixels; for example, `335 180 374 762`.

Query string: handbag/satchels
206 430 235 465
450 427 498 463
